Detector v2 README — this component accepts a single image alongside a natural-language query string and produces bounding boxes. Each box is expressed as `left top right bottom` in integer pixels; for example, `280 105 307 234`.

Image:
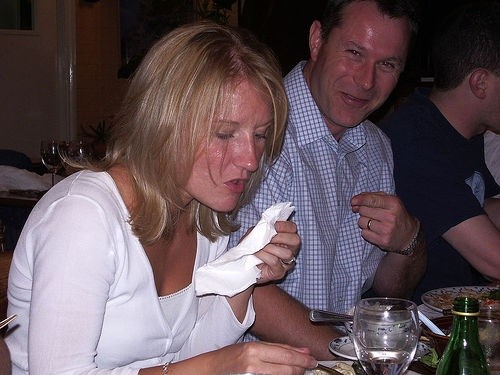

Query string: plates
304 361 422 375
421 286 500 322
328 336 430 361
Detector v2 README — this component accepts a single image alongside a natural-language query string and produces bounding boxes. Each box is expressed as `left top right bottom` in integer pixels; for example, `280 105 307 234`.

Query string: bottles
435 297 491 375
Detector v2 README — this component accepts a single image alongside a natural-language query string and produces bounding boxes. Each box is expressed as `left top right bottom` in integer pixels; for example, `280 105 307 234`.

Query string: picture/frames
117 0 197 79
0 0 39 36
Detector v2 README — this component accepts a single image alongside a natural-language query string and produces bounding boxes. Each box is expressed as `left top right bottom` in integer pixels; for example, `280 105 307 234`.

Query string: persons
377 11 500 304
5 19 318 375
228 0 426 363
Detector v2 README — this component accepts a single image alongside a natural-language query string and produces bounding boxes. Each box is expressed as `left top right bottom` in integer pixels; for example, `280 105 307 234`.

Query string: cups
353 297 419 375
70 135 92 174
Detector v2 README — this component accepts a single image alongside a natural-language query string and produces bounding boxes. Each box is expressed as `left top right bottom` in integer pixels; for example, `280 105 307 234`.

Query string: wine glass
40 136 67 188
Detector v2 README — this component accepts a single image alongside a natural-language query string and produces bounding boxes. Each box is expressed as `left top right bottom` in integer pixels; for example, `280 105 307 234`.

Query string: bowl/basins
344 314 413 348
421 316 500 357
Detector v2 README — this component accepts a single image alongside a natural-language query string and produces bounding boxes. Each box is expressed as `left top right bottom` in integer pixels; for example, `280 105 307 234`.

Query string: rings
368 218 375 230
282 257 296 264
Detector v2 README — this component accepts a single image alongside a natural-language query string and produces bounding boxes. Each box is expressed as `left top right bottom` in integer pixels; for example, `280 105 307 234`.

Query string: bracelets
392 217 424 255
161 362 170 375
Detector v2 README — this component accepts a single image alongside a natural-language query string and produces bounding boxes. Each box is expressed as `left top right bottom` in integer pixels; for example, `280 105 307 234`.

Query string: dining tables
332 275 500 375
